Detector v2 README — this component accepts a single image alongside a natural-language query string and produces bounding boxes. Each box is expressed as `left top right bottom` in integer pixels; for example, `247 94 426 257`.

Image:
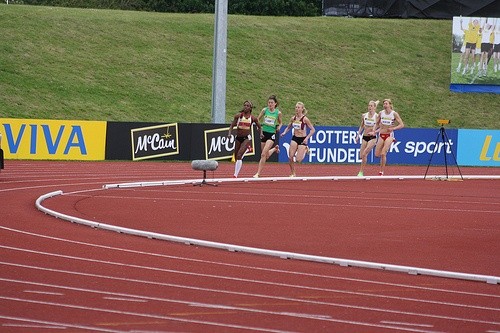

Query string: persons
373 99 404 175
355 100 381 176
456 14 500 77
228 100 261 178
252 94 280 177
280 102 315 177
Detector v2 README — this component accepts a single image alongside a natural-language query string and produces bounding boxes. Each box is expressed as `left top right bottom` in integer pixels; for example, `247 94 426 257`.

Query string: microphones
190 160 218 171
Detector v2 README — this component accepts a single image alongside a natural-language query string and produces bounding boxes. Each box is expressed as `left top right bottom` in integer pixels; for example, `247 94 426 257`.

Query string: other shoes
306 146 310 154
393 138 396 142
253 175 257 178
247 145 252 151
275 145 280 153
377 172 383 175
357 171 363 176
290 173 296 177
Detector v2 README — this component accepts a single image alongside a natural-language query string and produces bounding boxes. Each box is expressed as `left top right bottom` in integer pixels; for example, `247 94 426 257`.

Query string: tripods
423 124 463 180
